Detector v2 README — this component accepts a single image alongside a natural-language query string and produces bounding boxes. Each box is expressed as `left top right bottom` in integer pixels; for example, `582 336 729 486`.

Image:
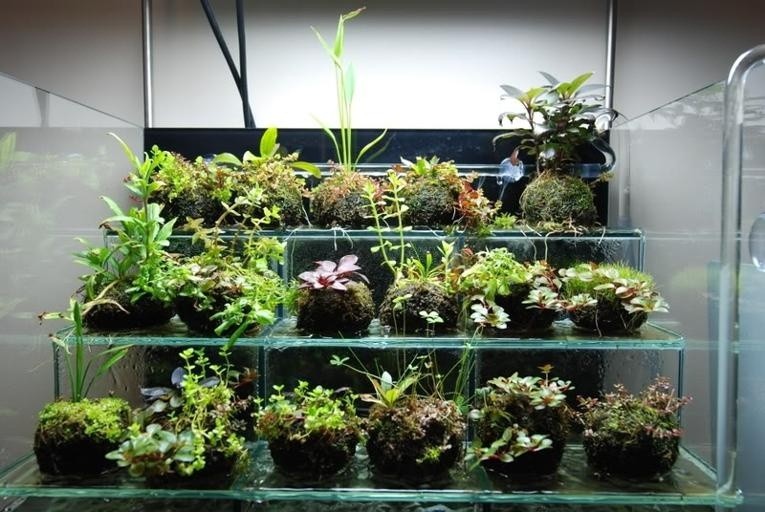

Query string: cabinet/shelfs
47 148 684 452
